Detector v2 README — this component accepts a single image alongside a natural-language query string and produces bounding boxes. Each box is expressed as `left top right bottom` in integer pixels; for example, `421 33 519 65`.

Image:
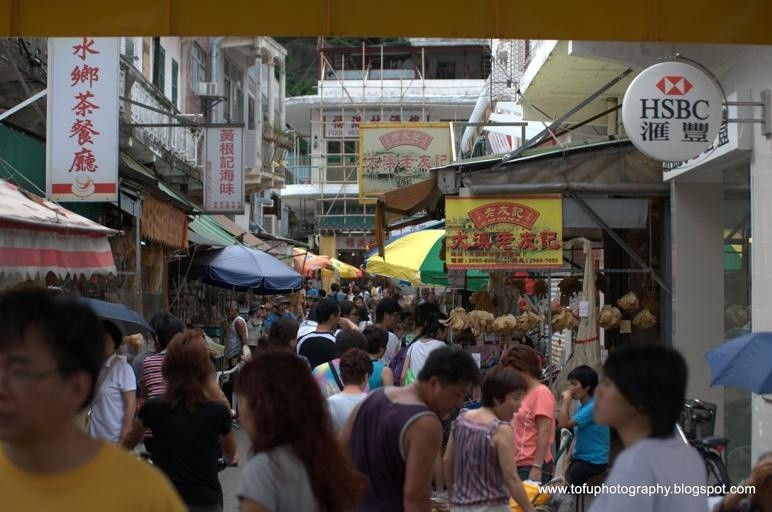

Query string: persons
723 229 744 270
1 281 708 512
713 448 772 510
705 331 771 395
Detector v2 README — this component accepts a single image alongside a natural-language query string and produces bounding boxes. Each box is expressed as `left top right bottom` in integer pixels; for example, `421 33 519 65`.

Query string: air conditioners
261 213 278 236
197 80 219 95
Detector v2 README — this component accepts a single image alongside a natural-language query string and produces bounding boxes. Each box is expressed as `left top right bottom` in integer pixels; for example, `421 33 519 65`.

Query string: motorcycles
510 329 557 387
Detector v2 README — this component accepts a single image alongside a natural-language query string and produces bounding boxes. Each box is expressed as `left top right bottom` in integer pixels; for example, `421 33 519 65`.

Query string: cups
75 176 94 192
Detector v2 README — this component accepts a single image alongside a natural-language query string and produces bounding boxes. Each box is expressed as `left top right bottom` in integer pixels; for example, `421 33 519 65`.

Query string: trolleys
509 475 565 512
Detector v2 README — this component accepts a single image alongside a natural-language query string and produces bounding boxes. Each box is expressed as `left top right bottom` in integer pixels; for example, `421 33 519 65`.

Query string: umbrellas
168 245 303 342
317 255 362 279
362 228 490 293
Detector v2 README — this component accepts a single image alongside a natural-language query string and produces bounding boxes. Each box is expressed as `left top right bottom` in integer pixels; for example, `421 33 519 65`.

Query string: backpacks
387 333 424 388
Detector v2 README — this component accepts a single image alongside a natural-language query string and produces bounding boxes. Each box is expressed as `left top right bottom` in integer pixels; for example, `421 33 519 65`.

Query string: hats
272 295 291 307
248 300 265 316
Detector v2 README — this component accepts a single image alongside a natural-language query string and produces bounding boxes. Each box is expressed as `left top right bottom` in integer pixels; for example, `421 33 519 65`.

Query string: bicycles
723 404 769 488
673 398 730 496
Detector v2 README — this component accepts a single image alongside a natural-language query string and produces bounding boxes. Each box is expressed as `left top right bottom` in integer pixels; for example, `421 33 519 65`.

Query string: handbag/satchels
79 406 93 437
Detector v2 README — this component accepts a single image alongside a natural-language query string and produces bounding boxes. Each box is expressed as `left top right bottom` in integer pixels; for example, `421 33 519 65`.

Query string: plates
73 183 95 198
